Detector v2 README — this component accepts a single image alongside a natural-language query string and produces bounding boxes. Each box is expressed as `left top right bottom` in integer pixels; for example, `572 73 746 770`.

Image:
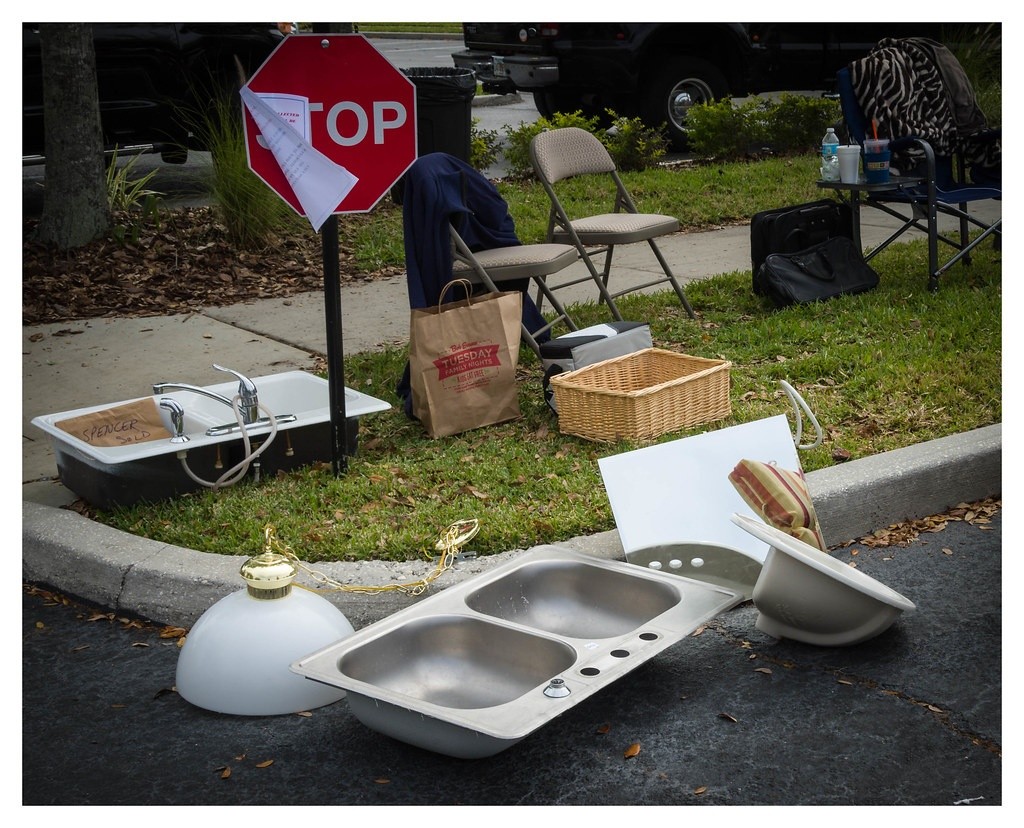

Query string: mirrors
597 414 830 601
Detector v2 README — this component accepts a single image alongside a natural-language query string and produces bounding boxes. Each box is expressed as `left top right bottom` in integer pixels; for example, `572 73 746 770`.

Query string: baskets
550 348 732 448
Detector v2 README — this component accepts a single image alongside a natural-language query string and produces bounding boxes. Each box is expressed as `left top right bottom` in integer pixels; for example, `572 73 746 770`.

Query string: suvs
452 22 919 152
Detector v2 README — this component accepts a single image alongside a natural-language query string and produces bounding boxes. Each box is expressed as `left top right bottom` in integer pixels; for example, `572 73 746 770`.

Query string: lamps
176 517 479 716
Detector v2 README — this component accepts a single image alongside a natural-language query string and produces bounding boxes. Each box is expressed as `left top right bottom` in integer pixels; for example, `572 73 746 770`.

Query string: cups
861 151 892 180
864 139 890 153
837 145 861 184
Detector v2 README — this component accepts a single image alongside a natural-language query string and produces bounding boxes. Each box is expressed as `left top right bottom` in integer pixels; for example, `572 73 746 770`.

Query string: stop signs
242 34 420 217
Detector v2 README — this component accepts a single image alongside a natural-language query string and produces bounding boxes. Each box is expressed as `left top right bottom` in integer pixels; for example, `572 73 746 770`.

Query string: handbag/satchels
751 198 880 312
410 279 522 440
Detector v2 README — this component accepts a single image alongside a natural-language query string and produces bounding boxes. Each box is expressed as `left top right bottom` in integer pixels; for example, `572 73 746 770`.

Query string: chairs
530 128 697 321
817 36 1002 291
403 153 579 361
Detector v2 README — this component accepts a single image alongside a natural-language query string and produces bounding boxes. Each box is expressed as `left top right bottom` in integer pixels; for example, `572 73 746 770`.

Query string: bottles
822 128 840 180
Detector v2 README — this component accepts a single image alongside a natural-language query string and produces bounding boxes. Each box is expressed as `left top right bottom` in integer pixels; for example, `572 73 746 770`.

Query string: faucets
151 382 259 424
159 398 191 443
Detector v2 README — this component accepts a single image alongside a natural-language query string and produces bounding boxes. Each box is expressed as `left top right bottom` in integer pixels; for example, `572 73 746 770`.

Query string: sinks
443 544 743 660
292 613 583 760
727 511 917 646
31 396 241 504
159 370 392 478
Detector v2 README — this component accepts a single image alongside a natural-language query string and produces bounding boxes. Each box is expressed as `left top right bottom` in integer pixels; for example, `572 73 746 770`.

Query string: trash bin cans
386 66 477 206
